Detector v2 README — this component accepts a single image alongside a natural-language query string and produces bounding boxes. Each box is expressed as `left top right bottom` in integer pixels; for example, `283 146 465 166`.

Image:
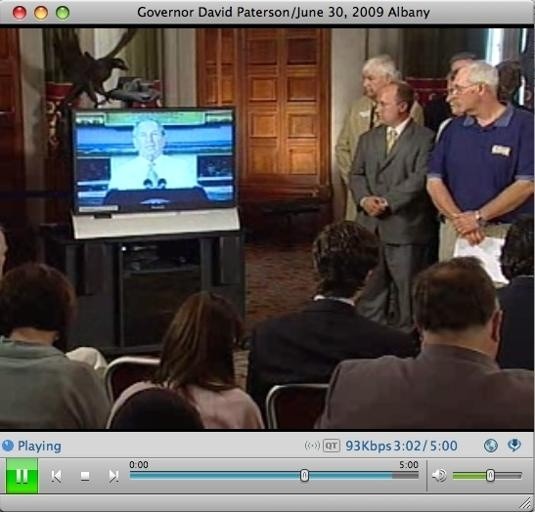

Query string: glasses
457 82 479 94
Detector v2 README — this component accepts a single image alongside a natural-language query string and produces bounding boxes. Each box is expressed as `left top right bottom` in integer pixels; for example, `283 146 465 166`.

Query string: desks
80 230 246 348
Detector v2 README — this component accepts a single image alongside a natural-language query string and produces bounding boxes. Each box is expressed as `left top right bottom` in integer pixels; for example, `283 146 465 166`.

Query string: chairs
494 60 521 105
102 354 331 431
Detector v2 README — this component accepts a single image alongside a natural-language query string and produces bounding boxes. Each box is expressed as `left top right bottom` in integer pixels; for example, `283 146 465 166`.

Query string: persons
109 387 204 431
314 256 534 432
335 52 533 368
245 220 420 430
105 290 265 430
108 118 200 191
1 263 113 431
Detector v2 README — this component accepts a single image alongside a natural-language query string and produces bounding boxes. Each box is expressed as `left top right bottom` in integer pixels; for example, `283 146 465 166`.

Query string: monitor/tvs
71 106 241 240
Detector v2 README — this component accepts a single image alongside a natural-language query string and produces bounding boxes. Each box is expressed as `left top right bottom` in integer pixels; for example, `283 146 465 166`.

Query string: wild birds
54 29 138 113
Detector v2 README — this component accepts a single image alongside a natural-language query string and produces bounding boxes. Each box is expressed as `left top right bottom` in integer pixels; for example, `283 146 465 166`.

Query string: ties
146 162 157 181
387 131 397 153
